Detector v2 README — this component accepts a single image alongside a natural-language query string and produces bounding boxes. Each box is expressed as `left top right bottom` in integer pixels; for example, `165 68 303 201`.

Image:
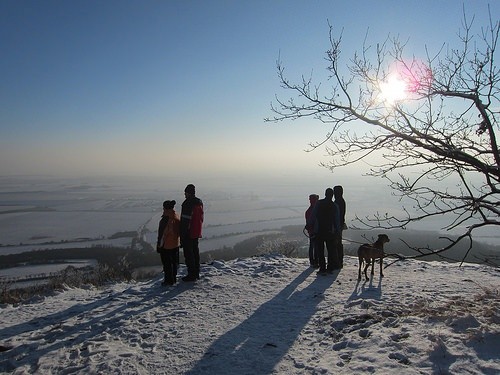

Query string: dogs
358 234 390 281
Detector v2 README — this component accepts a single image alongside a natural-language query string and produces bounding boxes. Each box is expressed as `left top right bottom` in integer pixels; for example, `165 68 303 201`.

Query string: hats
184 184 196 194
162 200 176 208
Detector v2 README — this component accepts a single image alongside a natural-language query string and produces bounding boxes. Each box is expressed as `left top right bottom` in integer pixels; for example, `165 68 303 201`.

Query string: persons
317 188 341 275
157 200 183 285
305 194 323 268
329 185 347 269
178 184 204 281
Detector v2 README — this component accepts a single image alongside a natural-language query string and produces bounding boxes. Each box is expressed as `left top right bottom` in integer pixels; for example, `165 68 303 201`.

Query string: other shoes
182 275 200 281
162 280 176 286
